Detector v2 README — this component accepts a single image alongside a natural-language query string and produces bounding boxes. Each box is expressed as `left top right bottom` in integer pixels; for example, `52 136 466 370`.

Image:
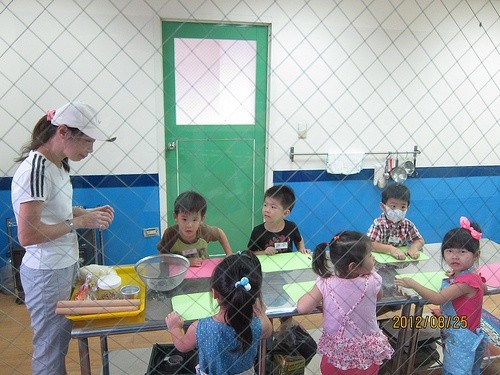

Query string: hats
50 102 116 143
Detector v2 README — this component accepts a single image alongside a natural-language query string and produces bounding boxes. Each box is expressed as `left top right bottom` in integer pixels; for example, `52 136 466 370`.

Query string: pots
390 159 408 184
400 160 415 176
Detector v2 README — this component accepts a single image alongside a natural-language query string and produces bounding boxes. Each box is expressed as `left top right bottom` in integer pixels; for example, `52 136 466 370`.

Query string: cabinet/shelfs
6 215 103 305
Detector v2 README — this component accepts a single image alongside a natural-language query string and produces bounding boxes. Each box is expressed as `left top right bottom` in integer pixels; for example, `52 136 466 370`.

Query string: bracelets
66 219 74 233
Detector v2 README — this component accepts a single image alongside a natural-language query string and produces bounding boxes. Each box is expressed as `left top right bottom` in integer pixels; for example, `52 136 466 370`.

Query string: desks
71 238 500 375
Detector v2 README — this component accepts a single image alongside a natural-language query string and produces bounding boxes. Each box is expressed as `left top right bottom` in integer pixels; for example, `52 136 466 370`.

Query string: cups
121 285 139 300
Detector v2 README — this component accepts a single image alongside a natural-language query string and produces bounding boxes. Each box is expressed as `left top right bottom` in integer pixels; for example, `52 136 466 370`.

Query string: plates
98 275 121 289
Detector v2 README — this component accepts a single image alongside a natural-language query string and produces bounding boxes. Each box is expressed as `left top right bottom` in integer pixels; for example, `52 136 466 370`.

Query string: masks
382 204 407 223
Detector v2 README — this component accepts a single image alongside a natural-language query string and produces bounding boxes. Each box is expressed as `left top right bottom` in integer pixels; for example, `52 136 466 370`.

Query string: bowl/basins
135 253 190 292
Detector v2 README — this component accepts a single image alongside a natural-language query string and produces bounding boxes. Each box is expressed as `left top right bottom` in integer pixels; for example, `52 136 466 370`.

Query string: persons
247 183 312 254
12 102 115 375
165 250 273 375
156 191 233 266
366 183 424 260
394 215 490 375
297 230 395 375
